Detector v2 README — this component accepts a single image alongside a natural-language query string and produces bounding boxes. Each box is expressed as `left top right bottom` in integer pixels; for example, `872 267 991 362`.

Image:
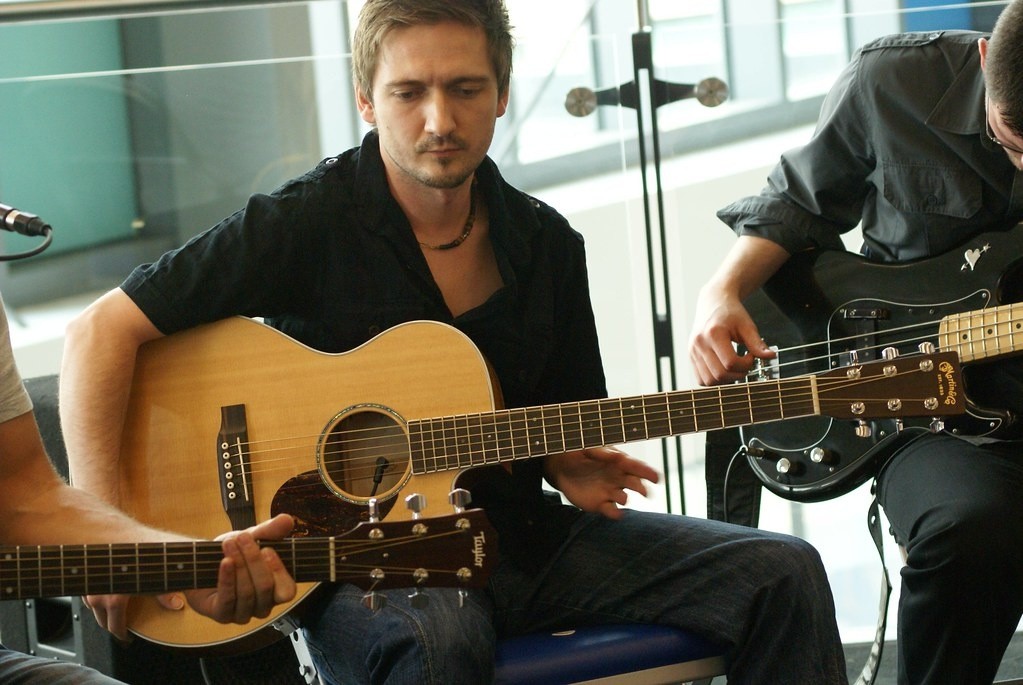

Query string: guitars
0 486 502 618
79 313 968 659
732 227 1023 505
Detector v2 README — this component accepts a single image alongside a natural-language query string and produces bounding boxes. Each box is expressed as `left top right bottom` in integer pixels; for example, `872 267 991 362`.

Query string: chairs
482 619 728 685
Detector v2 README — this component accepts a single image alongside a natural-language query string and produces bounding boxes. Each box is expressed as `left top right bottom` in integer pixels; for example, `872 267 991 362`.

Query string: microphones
0 202 52 237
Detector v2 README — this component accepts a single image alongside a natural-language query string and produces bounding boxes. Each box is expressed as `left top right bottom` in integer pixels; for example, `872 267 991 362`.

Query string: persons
0 295 301 685
688 3 1023 685
53 2 852 685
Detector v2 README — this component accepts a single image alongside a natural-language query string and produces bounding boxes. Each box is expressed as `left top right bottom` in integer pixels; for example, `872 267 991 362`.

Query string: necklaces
416 172 479 249
